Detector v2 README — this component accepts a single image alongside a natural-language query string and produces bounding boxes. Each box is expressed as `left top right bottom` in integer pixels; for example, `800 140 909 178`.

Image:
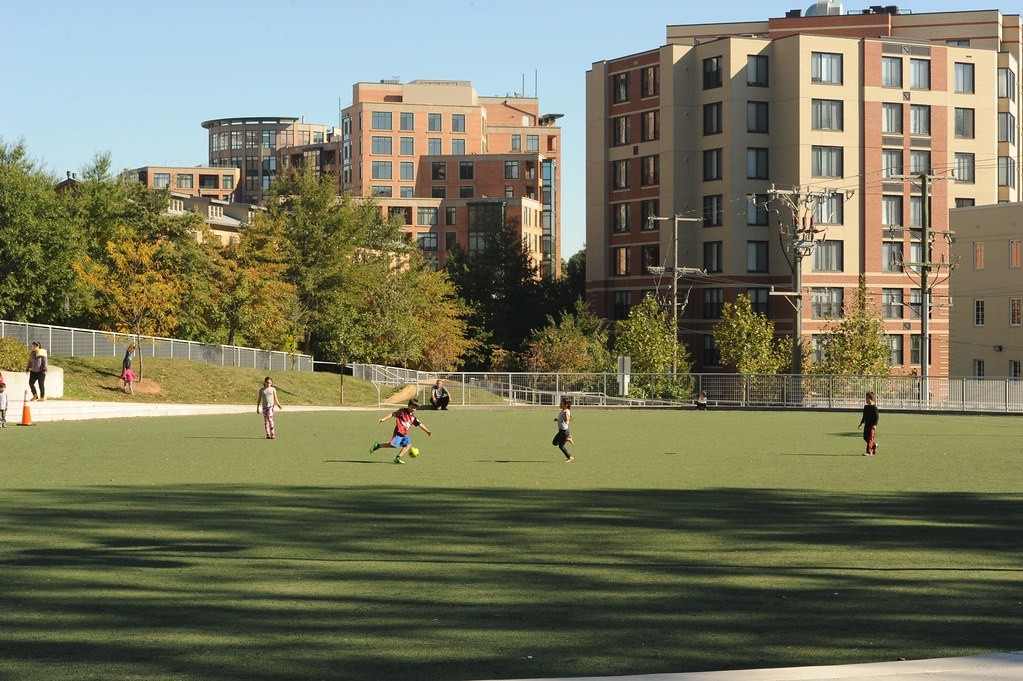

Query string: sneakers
369 440 381 453
393 455 406 464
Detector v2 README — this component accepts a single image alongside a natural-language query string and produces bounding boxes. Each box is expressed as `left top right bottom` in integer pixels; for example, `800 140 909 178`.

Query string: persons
119 361 137 396
0 372 8 428
26 341 48 402
696 390 707 411
257 377 282 439
430 380 452 410
552 398 574 463
122 342 136 380
369 399 433 465
858 392 879 456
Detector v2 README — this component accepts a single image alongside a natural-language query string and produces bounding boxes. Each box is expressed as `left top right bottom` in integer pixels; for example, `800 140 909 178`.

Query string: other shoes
267 436 275 439
29 393 45 402
565 455 575 463
862 443 880 456
567 435 574 444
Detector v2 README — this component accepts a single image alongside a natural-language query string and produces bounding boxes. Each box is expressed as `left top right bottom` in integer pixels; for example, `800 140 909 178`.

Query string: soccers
408 447 419 457
